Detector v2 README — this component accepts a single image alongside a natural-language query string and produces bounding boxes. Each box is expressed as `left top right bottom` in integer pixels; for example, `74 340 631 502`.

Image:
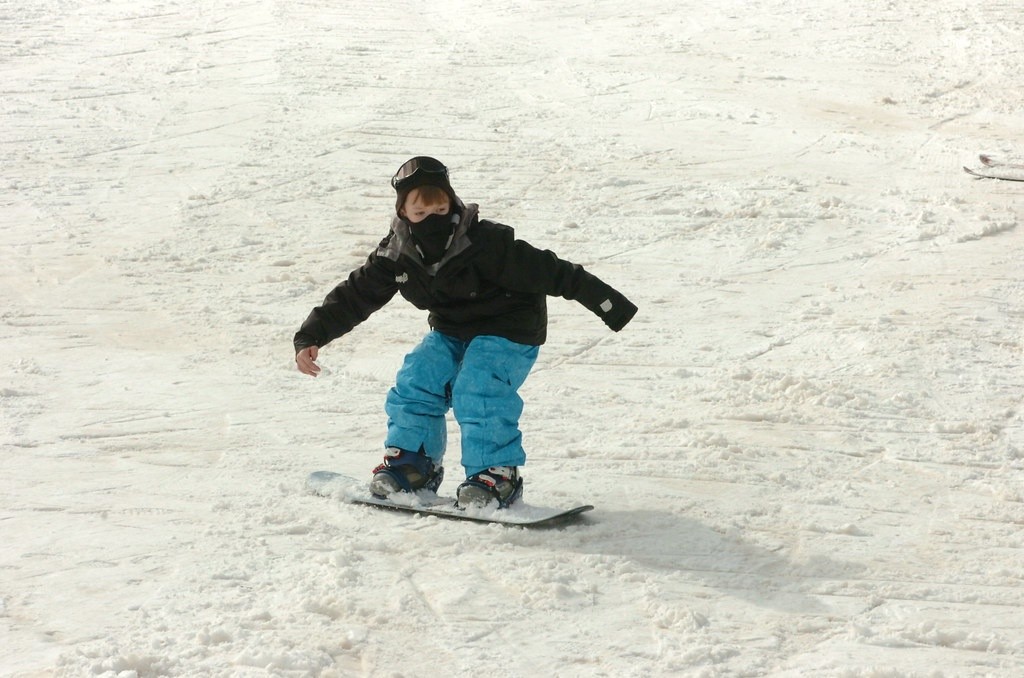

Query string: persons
292 156 639 511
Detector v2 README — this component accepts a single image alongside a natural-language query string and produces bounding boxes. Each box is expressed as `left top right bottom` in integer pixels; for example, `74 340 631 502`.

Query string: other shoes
372 448 444 496
459 464 522 509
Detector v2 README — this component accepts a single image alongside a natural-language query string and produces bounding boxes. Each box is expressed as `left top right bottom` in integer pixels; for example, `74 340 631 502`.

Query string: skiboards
962 153 1023 183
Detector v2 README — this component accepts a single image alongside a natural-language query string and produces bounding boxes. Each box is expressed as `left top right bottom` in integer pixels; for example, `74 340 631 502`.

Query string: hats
396 169 454 222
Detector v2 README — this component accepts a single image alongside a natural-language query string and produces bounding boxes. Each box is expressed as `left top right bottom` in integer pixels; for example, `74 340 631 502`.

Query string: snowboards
303 470 595 528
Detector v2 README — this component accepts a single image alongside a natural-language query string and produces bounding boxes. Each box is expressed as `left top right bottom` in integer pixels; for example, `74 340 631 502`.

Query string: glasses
391 157 448 190
